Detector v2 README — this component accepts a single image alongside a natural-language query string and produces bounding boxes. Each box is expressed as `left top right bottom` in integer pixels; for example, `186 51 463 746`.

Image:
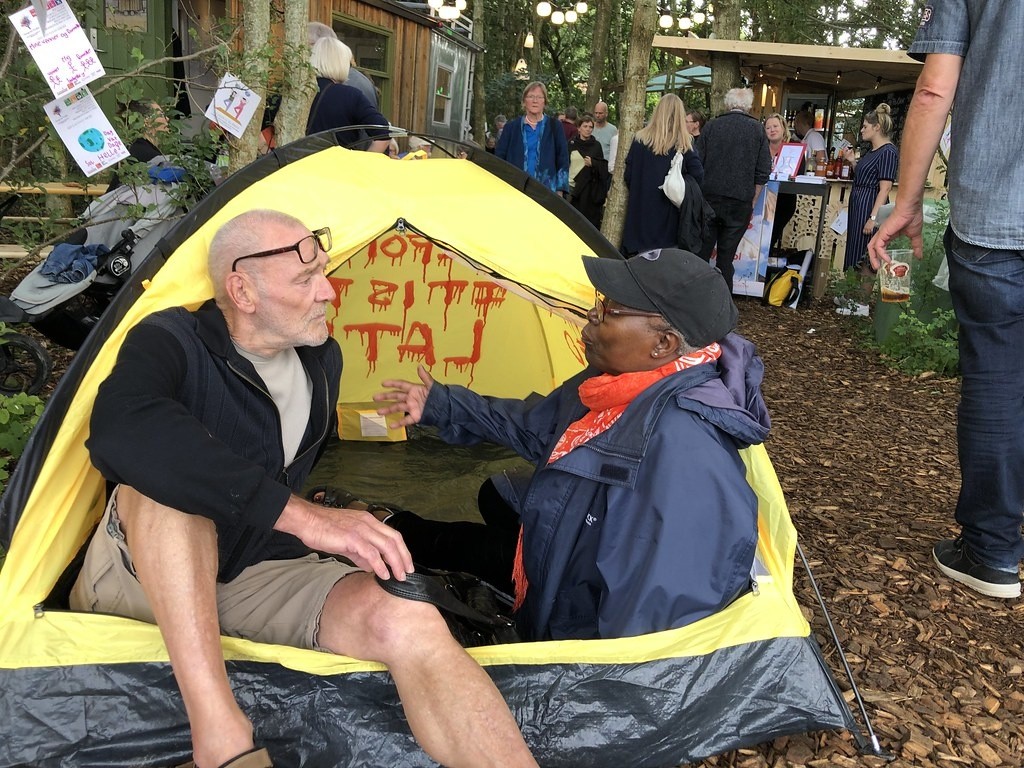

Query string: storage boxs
794 174 826 185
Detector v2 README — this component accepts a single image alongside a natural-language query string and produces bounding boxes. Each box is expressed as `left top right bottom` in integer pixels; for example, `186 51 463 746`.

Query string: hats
581 249 740 348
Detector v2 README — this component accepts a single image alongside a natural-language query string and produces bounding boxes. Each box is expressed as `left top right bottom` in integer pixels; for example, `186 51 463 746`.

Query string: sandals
306 486 403 526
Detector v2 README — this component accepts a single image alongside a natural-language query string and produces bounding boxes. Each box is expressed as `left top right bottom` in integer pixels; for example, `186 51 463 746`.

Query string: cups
814 108 824 129
878 249 914 303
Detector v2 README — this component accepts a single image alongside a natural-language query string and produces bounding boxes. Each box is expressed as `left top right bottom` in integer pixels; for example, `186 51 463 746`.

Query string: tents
0 124 882 768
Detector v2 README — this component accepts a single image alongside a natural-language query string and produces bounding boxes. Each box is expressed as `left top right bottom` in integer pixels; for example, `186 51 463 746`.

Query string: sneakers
933 533 1022 598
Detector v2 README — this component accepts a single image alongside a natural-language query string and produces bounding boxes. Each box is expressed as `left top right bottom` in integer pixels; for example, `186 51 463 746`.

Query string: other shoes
833 295 848 306
177 744 273 767
835 299 870 316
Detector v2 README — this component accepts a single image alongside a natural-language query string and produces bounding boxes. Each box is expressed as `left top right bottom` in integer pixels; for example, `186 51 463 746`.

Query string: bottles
216 144 230 167
826 151 834 178
787 110 792 128
841 159 850 180
834 150 843 179
804 150 816 176
815 157 826 177
791 110 797 128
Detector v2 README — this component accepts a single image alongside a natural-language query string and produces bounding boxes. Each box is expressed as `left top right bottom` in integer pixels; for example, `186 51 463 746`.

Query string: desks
765 179 831 310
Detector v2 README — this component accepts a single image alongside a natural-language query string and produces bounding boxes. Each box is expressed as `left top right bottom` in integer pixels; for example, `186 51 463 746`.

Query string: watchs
869 215 878 222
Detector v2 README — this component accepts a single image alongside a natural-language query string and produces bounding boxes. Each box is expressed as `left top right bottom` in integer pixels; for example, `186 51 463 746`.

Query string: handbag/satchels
659 150 685 207
761 265 803 307
372 560 520 646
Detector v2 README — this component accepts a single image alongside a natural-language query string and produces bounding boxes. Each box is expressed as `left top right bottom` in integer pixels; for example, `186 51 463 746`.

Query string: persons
103 21 899 317
867 0 1024 598
305 248 772 642
46 209 542 768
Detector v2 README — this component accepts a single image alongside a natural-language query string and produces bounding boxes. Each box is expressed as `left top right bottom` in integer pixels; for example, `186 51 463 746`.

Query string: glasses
525 94 545 100
593 290 664 323
232 227 334 273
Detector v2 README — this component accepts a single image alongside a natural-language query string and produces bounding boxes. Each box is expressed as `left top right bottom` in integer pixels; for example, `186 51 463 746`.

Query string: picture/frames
772 141 807 177
